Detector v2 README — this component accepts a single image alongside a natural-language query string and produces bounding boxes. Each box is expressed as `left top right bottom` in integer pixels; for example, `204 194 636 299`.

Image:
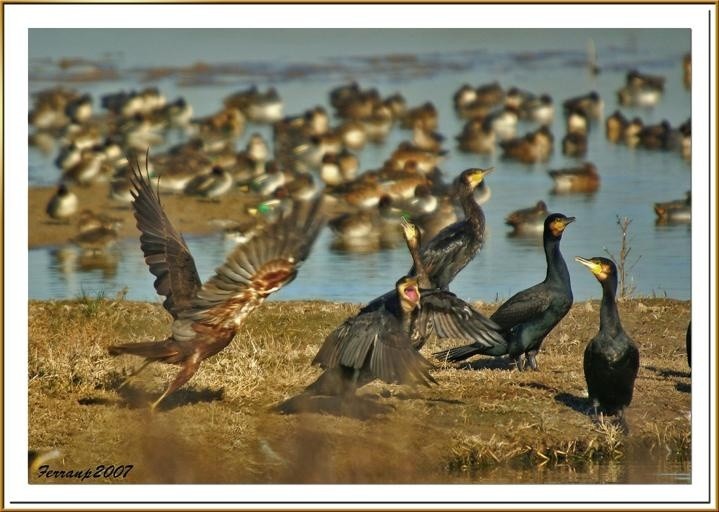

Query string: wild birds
307 276 440 396
575 254 640 415
108 144 331 416
434 213 578 374
28 70 692 257
400 215 505 351
405 165 494 289
